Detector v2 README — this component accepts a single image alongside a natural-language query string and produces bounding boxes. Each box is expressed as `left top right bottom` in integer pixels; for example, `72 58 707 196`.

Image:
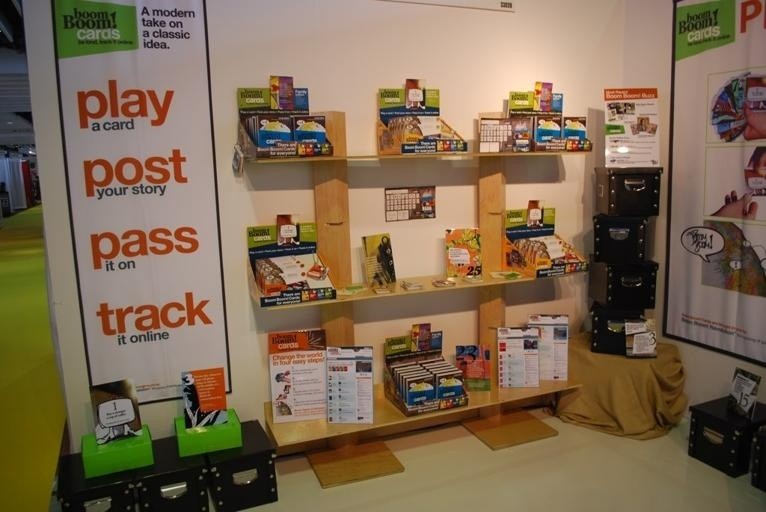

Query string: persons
710 191 758 220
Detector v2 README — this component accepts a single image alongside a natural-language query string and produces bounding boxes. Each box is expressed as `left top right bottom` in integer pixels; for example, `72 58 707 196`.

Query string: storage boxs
174 408 244 457
687 395 766 479
750 424 766 495
81 424 155 480
580 167 664 356
205 420 278 512
56 451 136 512
132 436 212 512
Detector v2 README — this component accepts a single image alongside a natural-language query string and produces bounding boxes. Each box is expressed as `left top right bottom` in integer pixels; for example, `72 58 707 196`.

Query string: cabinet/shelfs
229 114 593 490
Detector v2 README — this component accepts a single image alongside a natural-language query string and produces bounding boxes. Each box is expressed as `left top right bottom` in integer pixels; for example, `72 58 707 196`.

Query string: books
362 233 396 288
181 368 228 429
244 115 325 148
456 346 491 391
511 112 585 142
390 357 464 405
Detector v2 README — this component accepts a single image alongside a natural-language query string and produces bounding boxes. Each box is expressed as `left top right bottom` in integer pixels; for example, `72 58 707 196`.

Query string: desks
559 330 687 440
